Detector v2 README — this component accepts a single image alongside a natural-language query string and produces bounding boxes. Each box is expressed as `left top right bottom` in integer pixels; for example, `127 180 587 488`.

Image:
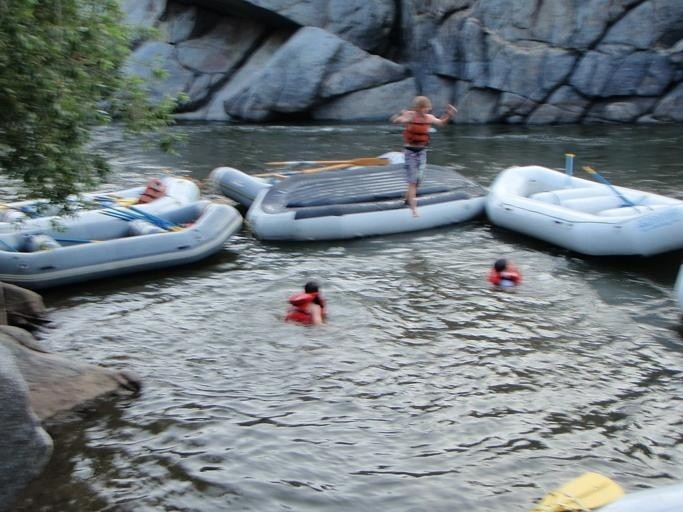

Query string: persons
489 261 520 286
387 95 458 216
285 282 325 326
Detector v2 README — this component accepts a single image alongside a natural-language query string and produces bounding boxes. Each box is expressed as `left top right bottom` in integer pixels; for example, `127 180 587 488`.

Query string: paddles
529 471 626 511
264 159 390 165
123 204 185 232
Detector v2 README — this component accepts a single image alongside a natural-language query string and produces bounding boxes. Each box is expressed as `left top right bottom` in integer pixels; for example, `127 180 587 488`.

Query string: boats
484 164 682 257
209 145 488 244
0 151 244 284
